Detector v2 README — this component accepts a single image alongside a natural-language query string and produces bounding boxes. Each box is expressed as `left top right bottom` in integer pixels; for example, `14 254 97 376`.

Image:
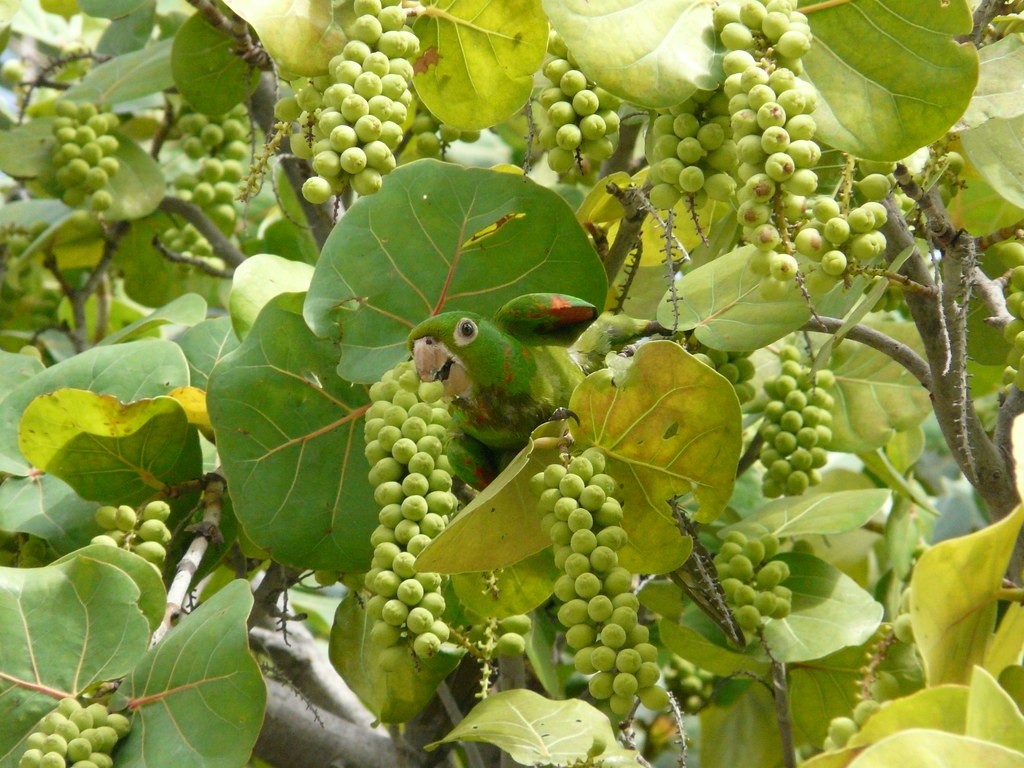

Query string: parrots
405 291 749 653
